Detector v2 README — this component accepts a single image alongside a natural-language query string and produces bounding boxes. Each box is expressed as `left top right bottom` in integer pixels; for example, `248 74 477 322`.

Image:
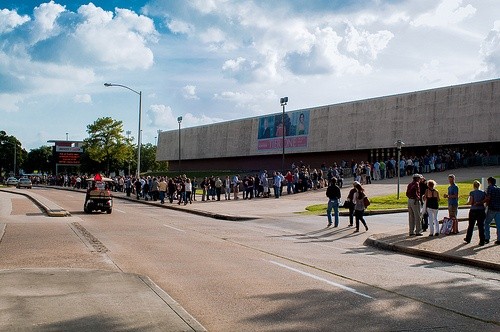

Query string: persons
104 184 110 195
326 177 341 227
200 170 271 201
400 148 490 177
351 158 396 185
273 161 327 197
347 181 369 232
444 174 458 232
474 177 500 245
464 180 487 246
91 182 98 190
109 174 198 206
29 173 88 189
405 174 423 236
419 175 428 232
425 180 440 236
327 159 348 188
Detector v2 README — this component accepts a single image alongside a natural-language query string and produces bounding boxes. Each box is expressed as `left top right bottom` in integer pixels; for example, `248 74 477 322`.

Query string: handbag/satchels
364 197 370 208
343 201 352 208
440 216 458 234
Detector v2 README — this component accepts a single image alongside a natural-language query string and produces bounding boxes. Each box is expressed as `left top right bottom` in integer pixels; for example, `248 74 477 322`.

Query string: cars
15 178 32 189
6 177 18 186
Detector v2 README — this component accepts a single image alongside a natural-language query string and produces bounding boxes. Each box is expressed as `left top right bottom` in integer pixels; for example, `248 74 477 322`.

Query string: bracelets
448 195 449 197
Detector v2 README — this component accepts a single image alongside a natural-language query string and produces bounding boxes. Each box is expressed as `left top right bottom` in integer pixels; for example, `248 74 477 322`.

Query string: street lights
394 139 403 199
279 96 288 174
104 82 143 176
177 115 183 175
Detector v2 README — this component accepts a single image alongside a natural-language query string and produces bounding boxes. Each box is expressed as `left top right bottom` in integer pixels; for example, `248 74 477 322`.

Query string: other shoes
354 229 359 233
409 234 415 236
429 234 433 236
126 194 197 205
479 243 484 246
334 225 337 227
258 187 321 199
348 224 353 226
485 239 489 243
365 226 368 230
495 241 500 245
201 196 257 201
416 233 423 236
435 233 439 236
327 223 331 226
464 238 470 243
